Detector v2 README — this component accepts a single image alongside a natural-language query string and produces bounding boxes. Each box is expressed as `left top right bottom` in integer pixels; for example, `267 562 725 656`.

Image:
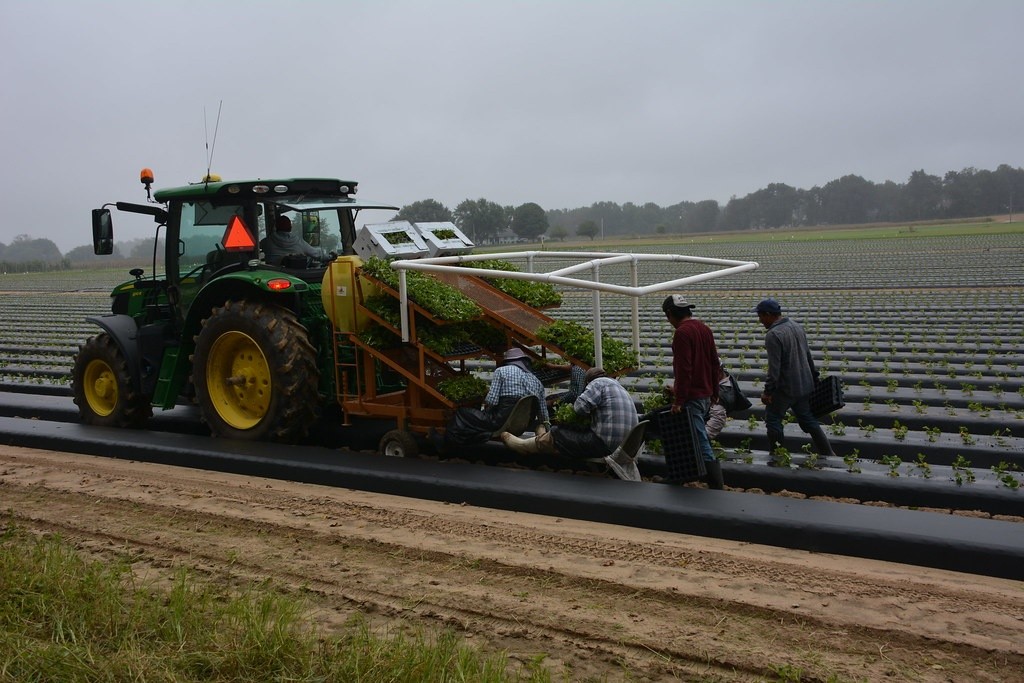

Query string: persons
501 367 639 457
704 357 733 438
430 348 550 459
663 294 723 489
547 366 586 415
241 216 337 269
750 299 836 455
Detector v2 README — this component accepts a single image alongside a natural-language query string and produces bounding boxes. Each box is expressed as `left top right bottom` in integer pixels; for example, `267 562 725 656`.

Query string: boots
535 424 546 436
500 430 559 457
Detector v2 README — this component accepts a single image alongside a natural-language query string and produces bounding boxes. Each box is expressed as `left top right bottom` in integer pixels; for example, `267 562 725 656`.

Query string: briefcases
657 407 707 484
809 375 845 418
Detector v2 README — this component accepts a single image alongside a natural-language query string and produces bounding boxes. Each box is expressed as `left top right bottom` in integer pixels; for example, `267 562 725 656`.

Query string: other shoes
431 425 446 453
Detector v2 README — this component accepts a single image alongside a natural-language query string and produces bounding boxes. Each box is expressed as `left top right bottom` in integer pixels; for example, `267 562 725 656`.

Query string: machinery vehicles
70 169 399 458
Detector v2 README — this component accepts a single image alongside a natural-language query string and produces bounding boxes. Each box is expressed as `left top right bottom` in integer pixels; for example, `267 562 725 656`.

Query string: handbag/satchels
715 372 752 412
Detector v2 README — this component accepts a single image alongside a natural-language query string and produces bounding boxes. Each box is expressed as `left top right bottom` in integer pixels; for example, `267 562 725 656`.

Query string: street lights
679 215 683 239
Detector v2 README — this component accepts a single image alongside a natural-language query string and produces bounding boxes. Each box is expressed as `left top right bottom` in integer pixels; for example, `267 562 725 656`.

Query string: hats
751 298 781 313
663 294 696 308
584 367 608 390
501 348 532 370
